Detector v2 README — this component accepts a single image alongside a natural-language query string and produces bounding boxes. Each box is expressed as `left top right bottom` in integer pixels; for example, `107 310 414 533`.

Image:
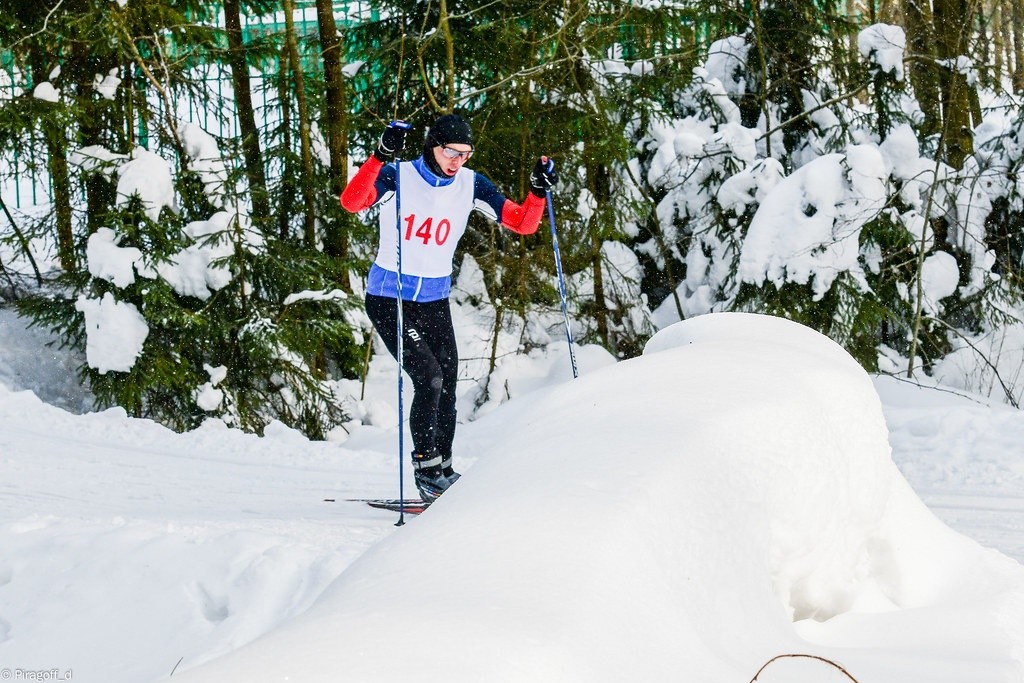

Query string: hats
426 115 474 148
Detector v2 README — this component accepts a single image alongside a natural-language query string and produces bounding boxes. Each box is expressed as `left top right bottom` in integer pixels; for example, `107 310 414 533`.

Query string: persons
340 112 560 503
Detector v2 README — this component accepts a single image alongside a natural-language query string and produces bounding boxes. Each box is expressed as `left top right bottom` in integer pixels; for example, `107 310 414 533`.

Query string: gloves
529 155 559 198
375 120 412 162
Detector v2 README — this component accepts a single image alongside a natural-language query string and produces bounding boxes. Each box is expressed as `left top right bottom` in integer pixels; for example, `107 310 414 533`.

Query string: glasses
442 146 475 160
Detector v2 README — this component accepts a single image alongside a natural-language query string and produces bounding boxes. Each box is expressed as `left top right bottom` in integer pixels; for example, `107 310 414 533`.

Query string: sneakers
435 450 462 484
411 448 451 504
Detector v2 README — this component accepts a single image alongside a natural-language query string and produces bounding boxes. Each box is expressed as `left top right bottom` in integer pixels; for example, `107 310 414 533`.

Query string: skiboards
326 497 437 515
